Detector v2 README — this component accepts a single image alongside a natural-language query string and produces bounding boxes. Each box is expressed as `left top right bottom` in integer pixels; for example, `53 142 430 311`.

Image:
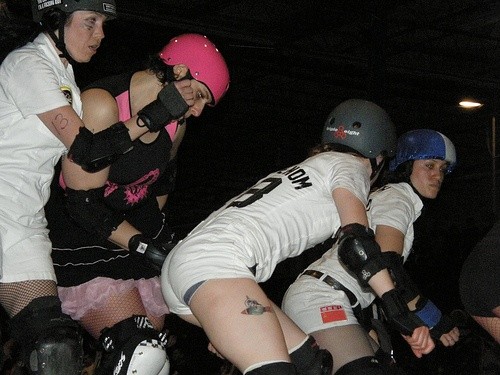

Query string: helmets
31 0 117 24
388 129 456 171
160 33 231 106
322 98 397 159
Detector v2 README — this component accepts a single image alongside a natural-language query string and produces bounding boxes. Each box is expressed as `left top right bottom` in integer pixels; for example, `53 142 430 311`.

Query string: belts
300 270 361 319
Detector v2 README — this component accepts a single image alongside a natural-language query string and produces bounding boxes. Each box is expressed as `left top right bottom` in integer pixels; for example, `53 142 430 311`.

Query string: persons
282 127 460 375
459 220 500 346
161 98 434 375
0 0 230 375
368 319 392 355
